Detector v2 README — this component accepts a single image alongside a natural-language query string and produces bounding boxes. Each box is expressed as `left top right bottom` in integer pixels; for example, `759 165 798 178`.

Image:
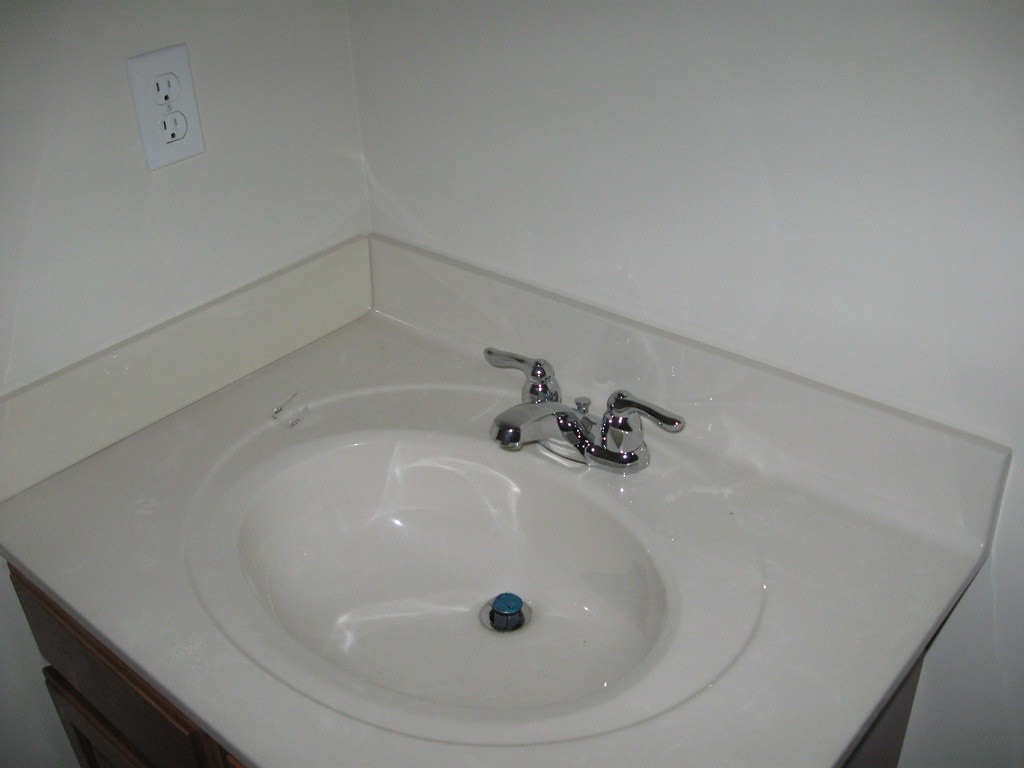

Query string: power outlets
123 42 205 171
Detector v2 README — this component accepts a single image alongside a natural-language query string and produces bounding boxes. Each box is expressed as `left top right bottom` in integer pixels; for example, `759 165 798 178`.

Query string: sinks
182 386 766 746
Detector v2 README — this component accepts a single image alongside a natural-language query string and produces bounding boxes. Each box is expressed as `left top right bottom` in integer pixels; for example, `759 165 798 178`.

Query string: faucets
483 346 685 474
